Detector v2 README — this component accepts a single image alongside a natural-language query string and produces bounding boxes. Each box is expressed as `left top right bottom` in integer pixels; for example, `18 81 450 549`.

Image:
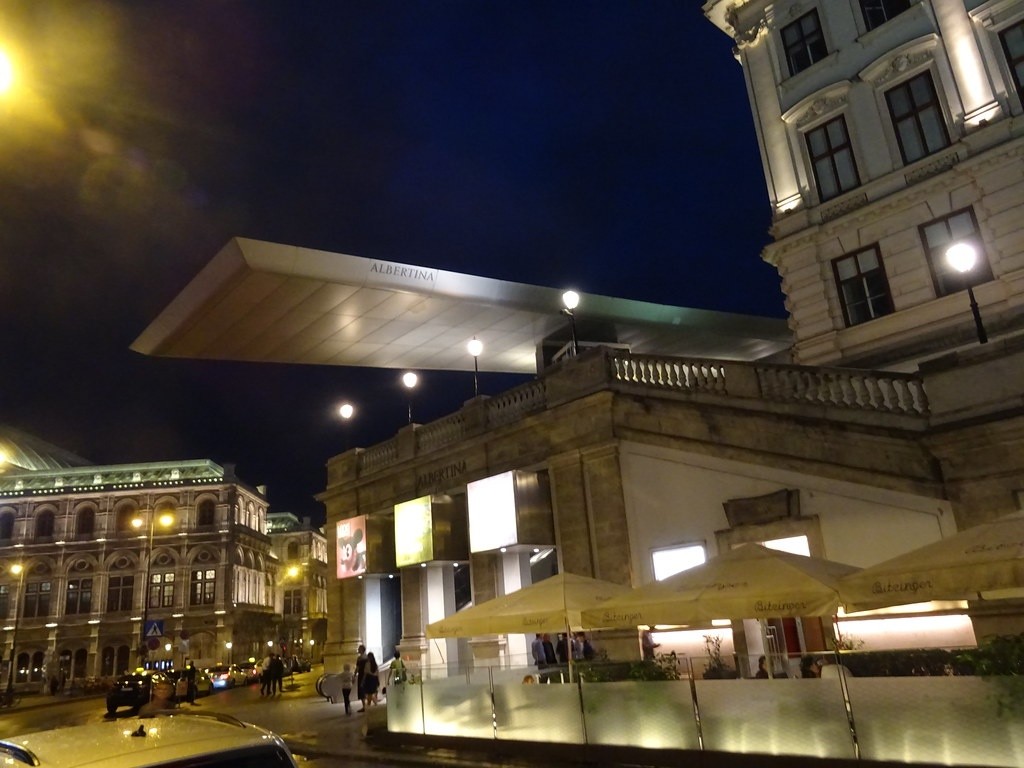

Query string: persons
43 672 66 697
523 632 596 684
340 644 408 716
757 654 855 722
188 660 196 702
641 626 660 660
260 653 284 695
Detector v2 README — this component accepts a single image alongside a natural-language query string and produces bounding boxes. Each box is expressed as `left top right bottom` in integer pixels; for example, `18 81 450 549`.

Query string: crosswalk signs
143 618 164 637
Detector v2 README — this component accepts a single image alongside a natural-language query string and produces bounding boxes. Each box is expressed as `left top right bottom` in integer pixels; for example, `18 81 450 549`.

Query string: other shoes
357 708 365 712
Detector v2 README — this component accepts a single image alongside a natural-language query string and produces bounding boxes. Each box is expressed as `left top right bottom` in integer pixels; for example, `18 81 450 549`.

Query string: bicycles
0 688 21 708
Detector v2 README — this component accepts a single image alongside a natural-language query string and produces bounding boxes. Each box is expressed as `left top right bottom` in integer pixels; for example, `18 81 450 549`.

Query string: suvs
0 710 301 768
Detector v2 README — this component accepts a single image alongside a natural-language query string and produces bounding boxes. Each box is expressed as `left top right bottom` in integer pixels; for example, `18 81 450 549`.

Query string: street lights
467 340 483 396
947 241 988 344
340 404 354 450
559 290 579 356
403 372 418 424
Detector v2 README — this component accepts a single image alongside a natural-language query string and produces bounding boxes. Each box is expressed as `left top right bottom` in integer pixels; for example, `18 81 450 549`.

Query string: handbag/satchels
401 659 408 681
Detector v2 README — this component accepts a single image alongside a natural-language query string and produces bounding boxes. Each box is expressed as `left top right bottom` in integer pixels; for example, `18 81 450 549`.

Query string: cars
206 664 248 689
238 662 257 680
142 670 173 688
298 658 311 672
106 673 154 712
254 659 264 676
176 673 213 698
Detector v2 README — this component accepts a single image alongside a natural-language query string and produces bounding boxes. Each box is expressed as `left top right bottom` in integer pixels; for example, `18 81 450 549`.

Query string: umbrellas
425 572 637 684
581 542 864 678
838 509 1024 615
